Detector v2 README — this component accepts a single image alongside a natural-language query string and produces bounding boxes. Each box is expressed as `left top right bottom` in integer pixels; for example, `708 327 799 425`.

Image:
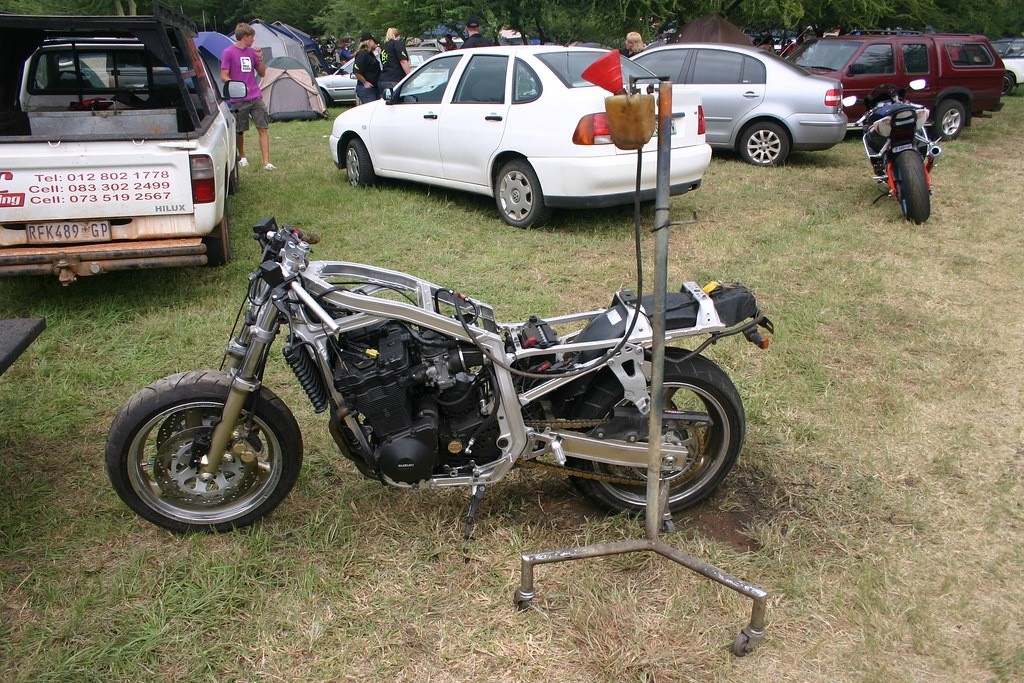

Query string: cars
314 45 451 108
567 39 850 169
328 42 714 229
407 36 624 98
972 37 1024 96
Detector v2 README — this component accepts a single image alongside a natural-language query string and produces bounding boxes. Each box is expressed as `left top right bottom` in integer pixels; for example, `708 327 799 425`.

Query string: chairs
467 78 501 102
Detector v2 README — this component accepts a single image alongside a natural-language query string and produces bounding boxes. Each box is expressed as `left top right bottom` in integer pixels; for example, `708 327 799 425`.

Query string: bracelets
362 81 367 85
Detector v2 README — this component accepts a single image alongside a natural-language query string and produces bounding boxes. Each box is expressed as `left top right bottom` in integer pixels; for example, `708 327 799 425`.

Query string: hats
466 18 480 28
359 32 378 42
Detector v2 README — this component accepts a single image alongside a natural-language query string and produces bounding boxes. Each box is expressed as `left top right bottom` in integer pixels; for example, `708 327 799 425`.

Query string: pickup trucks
0 12 250 290
775 29 1008 143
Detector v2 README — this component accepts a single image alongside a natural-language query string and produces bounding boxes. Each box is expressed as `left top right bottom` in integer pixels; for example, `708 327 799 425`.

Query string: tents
420 19 468 38
191 18 332 123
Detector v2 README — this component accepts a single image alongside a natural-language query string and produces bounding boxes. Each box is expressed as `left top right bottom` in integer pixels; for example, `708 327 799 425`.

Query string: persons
753 34 797 56
460 18 491 48
568 39 582 47
620 32 644 57
220 23 275 171
437 34 457 51
353 32 380 104
378 27 410 98
332 41 354 63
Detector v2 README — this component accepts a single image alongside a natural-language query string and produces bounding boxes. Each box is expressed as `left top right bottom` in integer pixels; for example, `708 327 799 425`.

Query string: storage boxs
28 105 178 134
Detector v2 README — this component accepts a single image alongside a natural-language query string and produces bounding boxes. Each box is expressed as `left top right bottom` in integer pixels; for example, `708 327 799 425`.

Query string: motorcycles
103 212 778 538
842 77 944 226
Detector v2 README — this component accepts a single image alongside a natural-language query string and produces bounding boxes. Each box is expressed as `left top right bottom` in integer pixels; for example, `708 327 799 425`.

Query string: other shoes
263 163 277 171
237 158 249 168
